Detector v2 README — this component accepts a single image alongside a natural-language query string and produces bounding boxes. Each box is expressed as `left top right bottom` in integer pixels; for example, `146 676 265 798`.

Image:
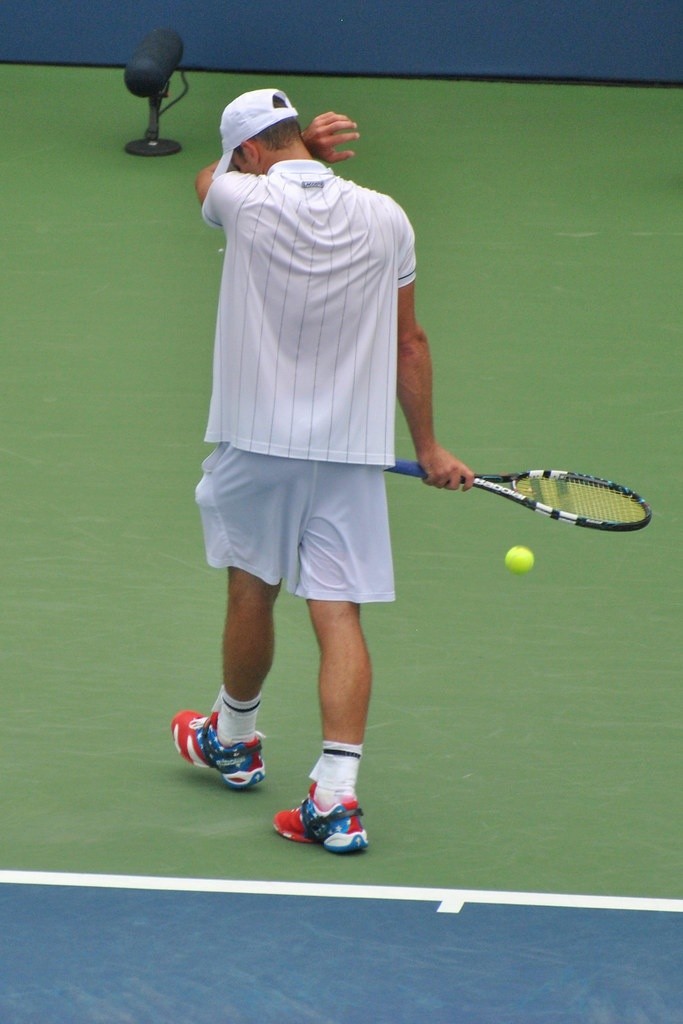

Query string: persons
169 87 475 851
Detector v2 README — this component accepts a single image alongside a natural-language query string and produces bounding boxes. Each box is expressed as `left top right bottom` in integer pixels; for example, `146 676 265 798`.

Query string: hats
211 89 299 180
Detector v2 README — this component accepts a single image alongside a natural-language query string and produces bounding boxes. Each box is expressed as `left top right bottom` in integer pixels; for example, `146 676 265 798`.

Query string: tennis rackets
384 457 653 533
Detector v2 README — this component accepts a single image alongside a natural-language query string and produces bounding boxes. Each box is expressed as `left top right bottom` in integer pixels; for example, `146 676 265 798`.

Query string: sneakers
273 784 368 852
172 710 265 788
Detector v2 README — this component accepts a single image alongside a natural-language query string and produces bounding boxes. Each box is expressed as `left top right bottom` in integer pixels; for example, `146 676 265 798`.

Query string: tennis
504 546 534 574
